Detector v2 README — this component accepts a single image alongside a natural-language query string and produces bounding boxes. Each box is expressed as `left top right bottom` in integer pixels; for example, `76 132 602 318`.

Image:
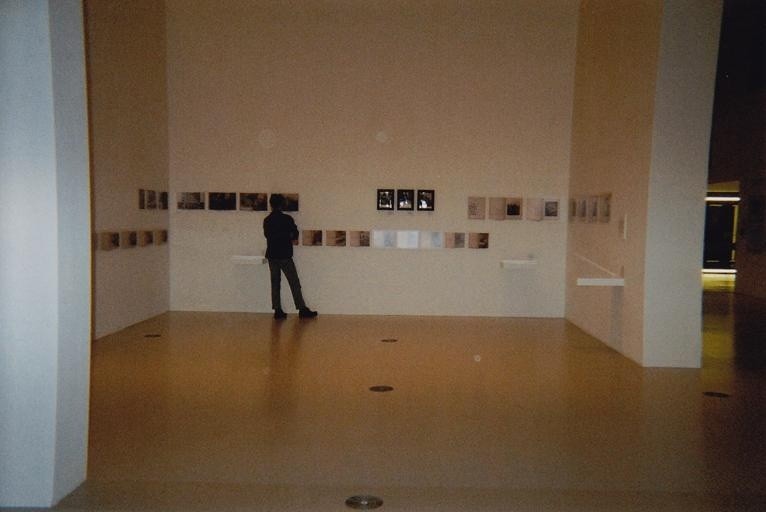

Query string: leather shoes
299 308 318 317
274 310 288 318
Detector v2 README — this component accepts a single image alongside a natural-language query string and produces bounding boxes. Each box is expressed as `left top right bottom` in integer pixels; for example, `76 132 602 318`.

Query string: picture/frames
397 189 414 210
377 189 394 210
543 200 560 219
417 189 434 211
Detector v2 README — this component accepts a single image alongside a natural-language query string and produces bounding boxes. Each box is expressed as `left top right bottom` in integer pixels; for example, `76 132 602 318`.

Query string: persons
261 193 318 320
